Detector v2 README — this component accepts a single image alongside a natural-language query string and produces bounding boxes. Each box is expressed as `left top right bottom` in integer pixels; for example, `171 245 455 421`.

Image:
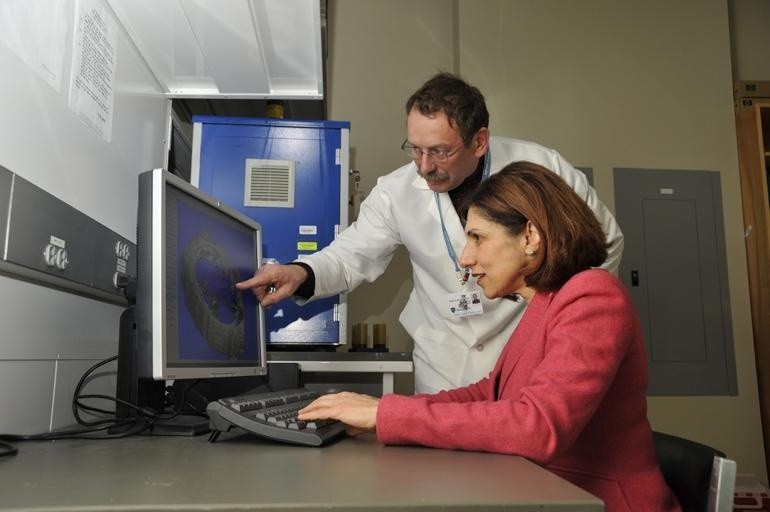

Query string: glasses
400 132 475 164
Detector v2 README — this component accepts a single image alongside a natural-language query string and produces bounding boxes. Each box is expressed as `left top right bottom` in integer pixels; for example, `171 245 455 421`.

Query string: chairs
649 428 736 512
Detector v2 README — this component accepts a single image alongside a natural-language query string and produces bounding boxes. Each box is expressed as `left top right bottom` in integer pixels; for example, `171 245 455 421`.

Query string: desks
0 349 606 512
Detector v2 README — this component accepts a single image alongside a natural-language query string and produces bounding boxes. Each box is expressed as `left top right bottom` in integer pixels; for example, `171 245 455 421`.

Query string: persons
232 70 629 399
293 161 680 511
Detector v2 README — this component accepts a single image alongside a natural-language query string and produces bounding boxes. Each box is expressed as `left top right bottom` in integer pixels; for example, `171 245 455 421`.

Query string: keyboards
205 386 358 447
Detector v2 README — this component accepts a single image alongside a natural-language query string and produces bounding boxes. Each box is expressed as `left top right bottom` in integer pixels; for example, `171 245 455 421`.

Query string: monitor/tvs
109 169 268 436
